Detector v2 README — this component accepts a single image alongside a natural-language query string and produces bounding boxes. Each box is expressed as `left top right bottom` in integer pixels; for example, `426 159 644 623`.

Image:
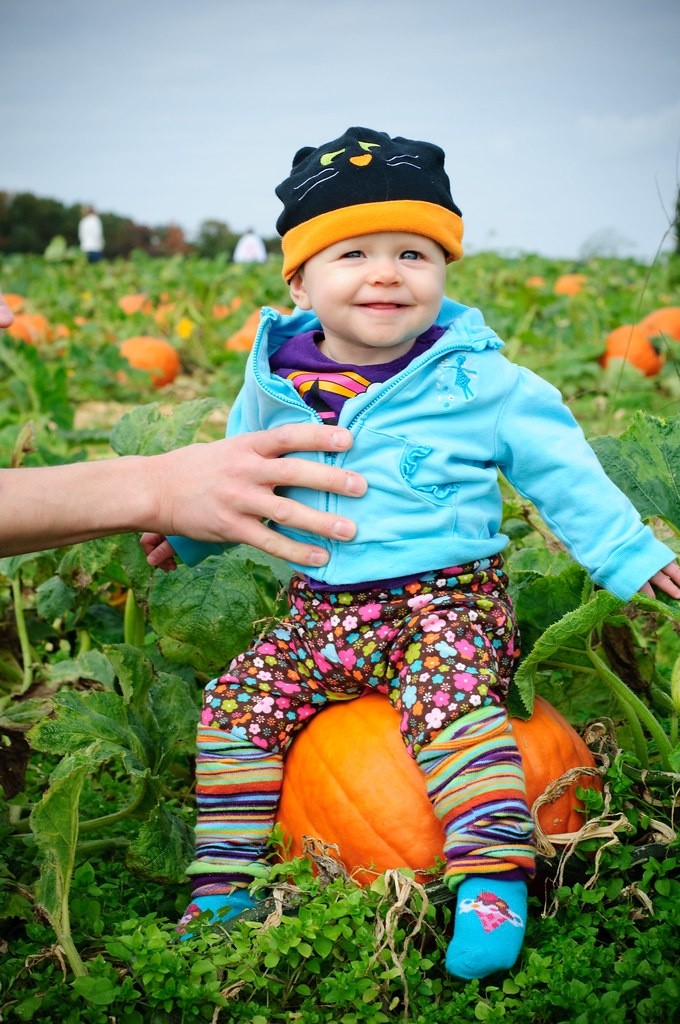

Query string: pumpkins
521 272 680 376
265 685 603 896
0 292 295 390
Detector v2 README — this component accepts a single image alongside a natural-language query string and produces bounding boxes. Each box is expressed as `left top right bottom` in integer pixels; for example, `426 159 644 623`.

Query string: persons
232 226 268 263
0 422 366 567
141 128 680 980
79 204 105 263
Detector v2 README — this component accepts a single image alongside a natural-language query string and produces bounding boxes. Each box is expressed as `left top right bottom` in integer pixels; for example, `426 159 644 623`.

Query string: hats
274 127 464 286
